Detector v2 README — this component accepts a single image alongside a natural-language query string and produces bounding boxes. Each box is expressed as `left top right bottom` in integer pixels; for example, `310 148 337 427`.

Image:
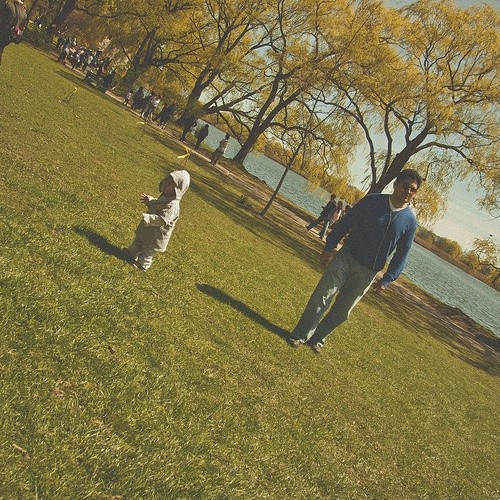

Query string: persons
55 29 231 167
121 170 190 273
0 0 28 64
286 168 423 354
304 192 352 245
